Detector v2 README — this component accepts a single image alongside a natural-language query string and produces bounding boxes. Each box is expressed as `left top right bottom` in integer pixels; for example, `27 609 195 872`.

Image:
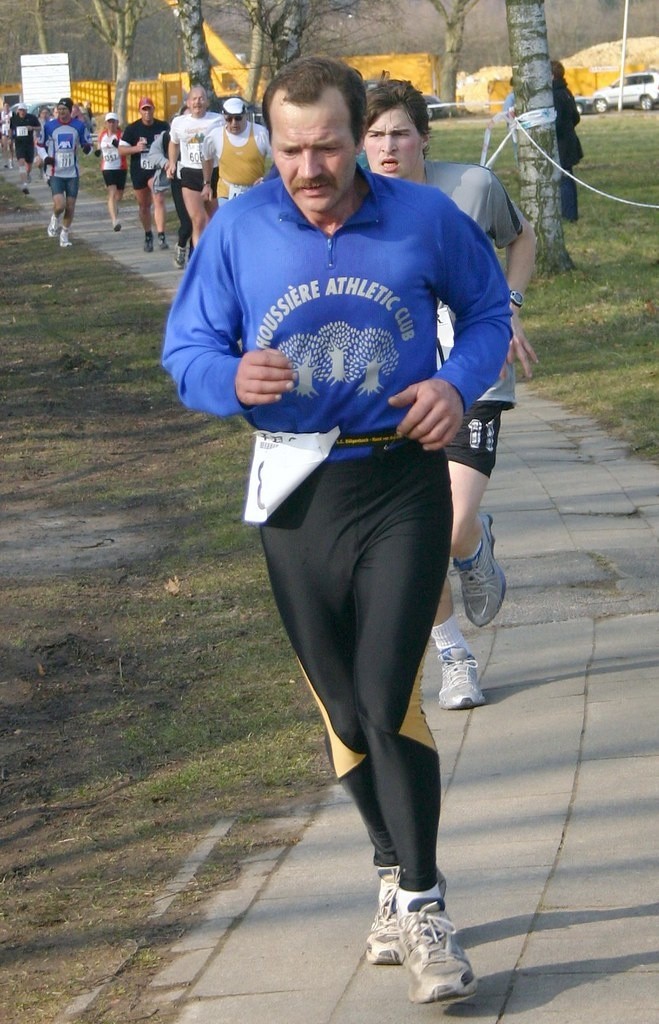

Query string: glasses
142 106 152 111
223 113 245 122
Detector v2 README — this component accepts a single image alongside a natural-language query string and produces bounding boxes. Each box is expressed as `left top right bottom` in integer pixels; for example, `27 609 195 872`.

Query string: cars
504 92 592 130
0 103 34 136
30 102 90 146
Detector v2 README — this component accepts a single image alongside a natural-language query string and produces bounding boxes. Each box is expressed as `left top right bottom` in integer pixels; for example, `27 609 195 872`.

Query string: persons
167 87 226 248
362 77 536 710
202 97 280 205
118 99 171 251
503 78 518 168
163 61 514 1003
148 106 194 268
95 113 128 232
551 61 584 222
0 98 93 247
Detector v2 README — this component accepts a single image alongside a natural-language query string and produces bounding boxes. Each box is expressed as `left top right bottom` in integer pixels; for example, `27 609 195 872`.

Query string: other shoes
25 174 31 183
3 161 13 170
47 179 51 187
23 185 29 194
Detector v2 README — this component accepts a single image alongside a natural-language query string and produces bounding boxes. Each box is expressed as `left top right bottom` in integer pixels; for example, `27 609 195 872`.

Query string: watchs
510 290 524 308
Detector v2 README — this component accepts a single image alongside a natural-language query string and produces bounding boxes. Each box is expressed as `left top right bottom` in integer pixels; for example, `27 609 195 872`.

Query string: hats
222 97 247 117
139 98 154 111
18 103 27 109
58 98 73 113
105 113 118 121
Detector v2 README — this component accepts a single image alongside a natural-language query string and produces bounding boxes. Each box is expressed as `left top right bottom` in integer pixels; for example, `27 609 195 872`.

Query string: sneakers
366 861 446 964
449 514 506 628
395 898 477 1006
173 241 186 268
113 222 121 232
60 231 72 248
437 647 485 710
158 235 169 248
144 236 153 252
48 223 58 238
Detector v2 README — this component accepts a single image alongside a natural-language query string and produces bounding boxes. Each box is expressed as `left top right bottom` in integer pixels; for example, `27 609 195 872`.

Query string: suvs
593 72 659 112
364 79 445 120
168 96 265 133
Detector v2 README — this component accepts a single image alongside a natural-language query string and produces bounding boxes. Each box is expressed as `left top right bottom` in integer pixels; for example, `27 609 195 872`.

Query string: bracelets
203 181 210 185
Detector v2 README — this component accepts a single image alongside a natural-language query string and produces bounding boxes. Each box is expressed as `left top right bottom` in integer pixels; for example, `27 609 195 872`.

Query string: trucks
463 62 659 116
339 52 444 98
3 93 21 108
69 71 190 125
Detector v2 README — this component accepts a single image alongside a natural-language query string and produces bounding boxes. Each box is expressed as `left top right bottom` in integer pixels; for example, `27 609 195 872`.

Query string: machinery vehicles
158 0 273 116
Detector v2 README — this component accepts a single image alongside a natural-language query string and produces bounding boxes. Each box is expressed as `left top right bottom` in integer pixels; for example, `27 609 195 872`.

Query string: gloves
45 157 51 164
82 144 90 154
95 150 101 157
112 139 118 147
